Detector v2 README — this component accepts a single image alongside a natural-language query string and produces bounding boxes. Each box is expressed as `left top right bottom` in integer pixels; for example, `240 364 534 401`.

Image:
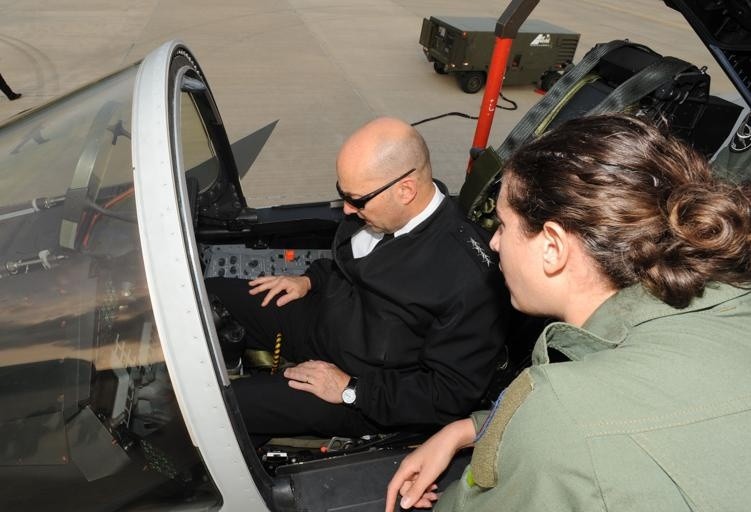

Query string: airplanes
0 1 750 512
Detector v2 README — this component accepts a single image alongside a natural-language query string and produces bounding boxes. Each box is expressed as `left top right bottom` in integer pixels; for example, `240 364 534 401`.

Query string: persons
231 117 507 448
385 116 751 512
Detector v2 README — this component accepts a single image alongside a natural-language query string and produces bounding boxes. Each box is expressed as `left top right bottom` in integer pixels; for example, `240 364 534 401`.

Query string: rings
307 375 309 383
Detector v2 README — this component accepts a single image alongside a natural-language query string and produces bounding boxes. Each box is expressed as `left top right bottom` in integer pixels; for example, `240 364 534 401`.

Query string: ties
372 233 394 250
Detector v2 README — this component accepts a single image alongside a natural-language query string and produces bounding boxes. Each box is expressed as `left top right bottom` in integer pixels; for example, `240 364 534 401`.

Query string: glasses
336 168 416 210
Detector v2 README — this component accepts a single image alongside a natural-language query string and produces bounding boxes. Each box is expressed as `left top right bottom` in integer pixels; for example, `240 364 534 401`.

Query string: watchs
341 376 358 407
